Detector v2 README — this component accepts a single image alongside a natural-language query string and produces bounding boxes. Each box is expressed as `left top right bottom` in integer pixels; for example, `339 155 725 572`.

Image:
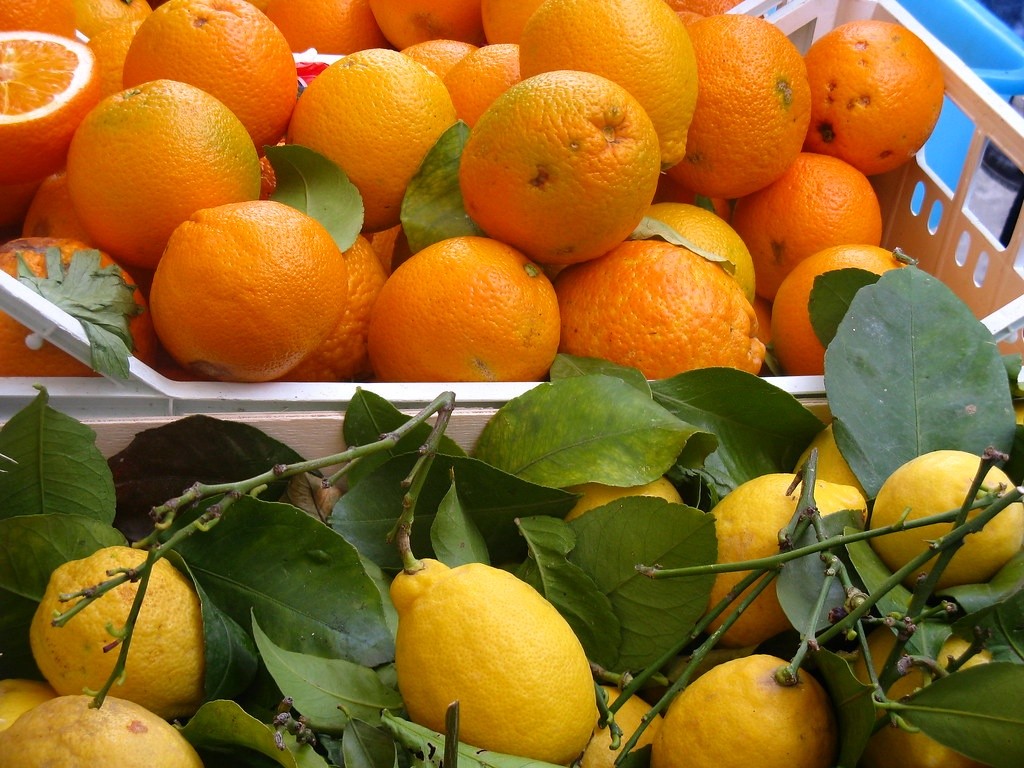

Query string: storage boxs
0 0 1024 484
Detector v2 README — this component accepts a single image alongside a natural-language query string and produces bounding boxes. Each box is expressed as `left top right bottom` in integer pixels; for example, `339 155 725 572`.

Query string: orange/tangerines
0 0 943 381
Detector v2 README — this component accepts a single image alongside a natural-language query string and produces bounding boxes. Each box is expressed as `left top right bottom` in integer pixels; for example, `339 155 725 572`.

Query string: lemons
0 414 1024 768
